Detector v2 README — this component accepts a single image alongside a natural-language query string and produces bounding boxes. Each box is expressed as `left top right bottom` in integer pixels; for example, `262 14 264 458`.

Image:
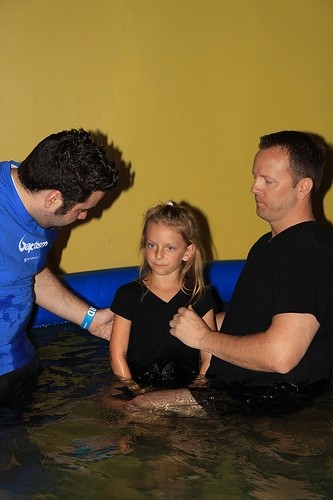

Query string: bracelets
80 306 97 330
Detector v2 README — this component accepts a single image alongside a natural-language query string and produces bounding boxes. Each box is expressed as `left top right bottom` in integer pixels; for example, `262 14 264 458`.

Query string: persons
132 131 333 407
0 129 120 376
109 201 216 377
